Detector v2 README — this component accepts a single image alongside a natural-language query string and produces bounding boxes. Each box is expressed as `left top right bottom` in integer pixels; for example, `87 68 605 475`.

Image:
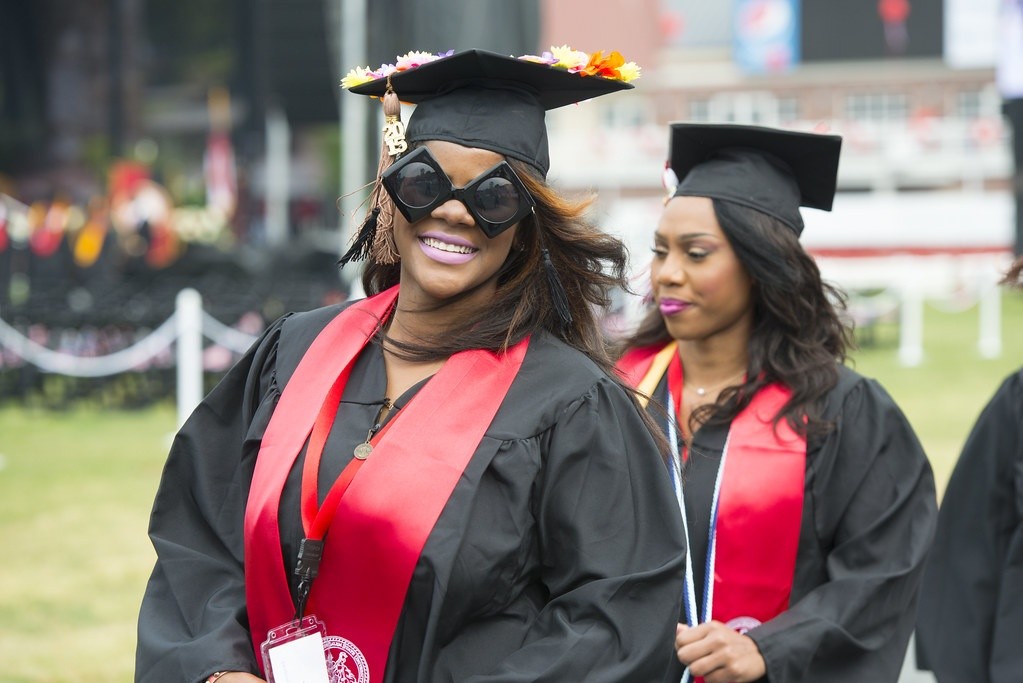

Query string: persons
608 123 939 683
133 44 687 683
914 258 1023 683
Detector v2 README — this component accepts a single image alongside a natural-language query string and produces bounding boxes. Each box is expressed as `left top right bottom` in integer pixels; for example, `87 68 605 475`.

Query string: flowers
339 44 642 107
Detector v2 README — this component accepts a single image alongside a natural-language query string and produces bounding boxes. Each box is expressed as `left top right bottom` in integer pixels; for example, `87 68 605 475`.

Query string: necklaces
684 369 747 395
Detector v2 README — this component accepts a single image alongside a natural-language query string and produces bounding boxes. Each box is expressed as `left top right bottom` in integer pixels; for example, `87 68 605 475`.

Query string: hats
662 123 842 235
338 49 635 273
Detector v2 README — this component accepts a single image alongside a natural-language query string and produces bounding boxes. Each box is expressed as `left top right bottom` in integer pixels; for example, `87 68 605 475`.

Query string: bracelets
205 671 227 683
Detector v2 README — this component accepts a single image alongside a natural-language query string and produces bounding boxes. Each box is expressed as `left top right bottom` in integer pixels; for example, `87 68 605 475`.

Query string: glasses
379 144 536 239
